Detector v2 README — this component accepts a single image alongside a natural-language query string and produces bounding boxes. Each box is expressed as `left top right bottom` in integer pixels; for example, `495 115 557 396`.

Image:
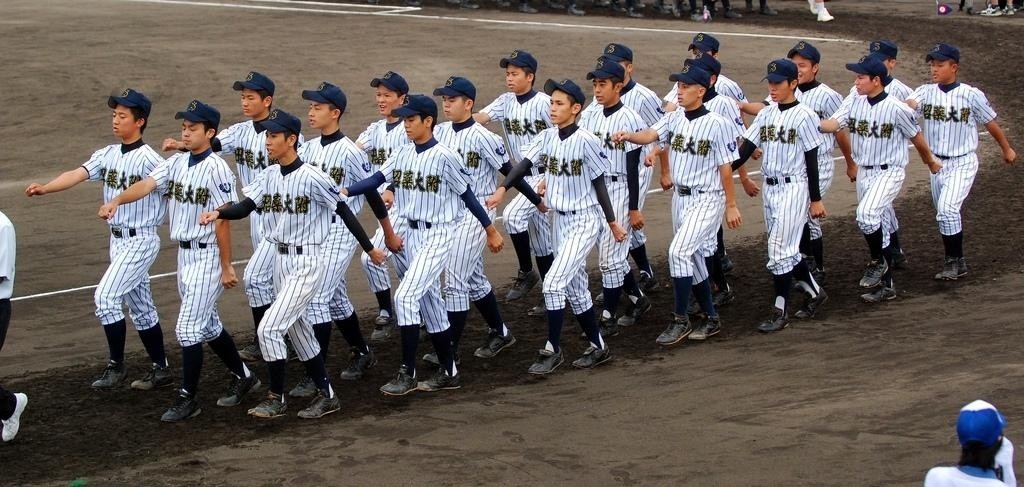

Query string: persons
0 209 29 442
808 1 834 24
956 0 1023 18
26 34 764 423
402 1 780 24
921 399 1018 487
730 40 1014 335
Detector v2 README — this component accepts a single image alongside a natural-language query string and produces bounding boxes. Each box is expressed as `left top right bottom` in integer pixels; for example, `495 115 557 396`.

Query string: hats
955 399 1008 449
109 87 151 116
301 82 347 114
433 77 476 100
925 43 959 63
669 33 721 89
787 42 820 63
586 43 633 83
232 71 274 97
257 108 301 137
500 49 537 71
544 78 586 106
390 93 437 119
369 71 408 95
176 100 221 127
845 40 898 80
759 59 798 83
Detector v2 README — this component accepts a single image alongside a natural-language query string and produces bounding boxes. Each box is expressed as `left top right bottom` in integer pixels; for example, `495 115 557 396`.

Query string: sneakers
340 347 379 380
253 390 287 418
758 5 779 17
859 248 908 303
593 0 610 7
379 350 461 396
744 3 753 13
130 358 174 392
571 342 612 369
528 346 565 374
527 297 570 317
238 337 299 362
546 0 566 10
217 369 262 407
980 4 1002 17
367 0 385 4
403 1 422 7
444 1 481 10
579 275 661 340
935 255 969 281
496 1 511 8
160 387 201 423
1000 6 1015 15
656 255 734 346
723 4 742 19
691 8 705 21
817 8 835 22
90 360 130 391
652 2 689 18
757 305 789 332
808 0 819 15
2 392 28 442
610 1 647 18
517 3 538 14
567 4 587 16
296 392 342 419
506 269 540 300
289 370 319 398
371 315 397 345
473 329 517 359
794 256 828 322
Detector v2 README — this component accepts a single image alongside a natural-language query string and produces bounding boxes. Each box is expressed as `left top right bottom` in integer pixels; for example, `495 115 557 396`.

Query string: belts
766 177 790 185
407 218 432 229
180 239 206 249
673 185 704 196
862 165 889 170
935 154 964 160
526 167 545 176
557 210 574 216
612 175 616 180
278 242 302 254
111 227 136 239
